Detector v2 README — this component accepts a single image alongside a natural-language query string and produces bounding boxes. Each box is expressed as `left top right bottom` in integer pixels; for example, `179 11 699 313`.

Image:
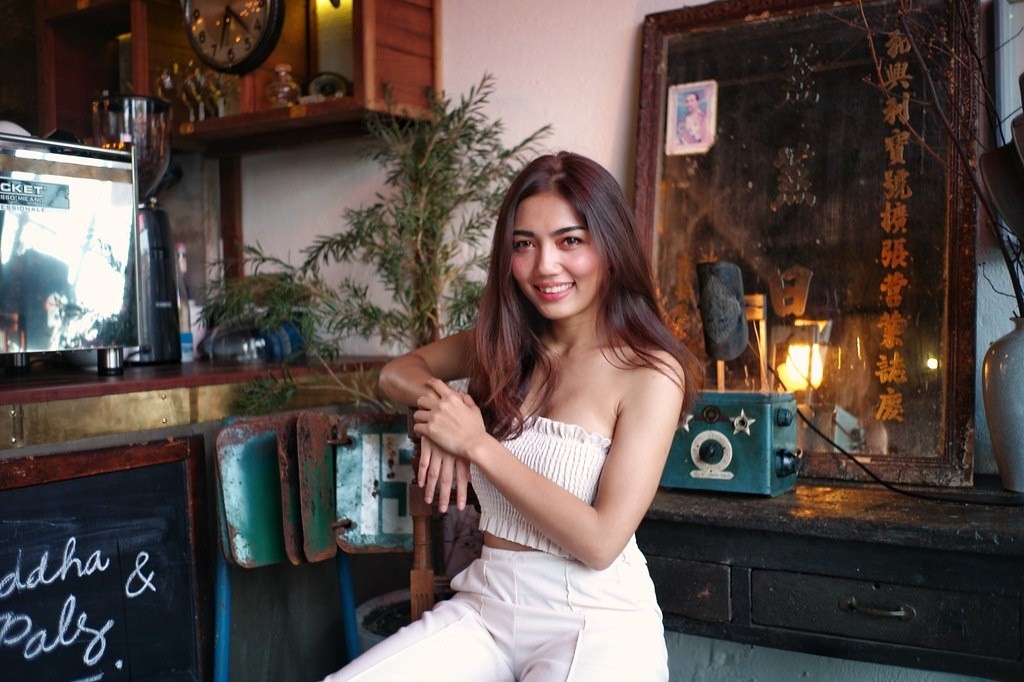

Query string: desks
0 354 394 682
469 479 1024 682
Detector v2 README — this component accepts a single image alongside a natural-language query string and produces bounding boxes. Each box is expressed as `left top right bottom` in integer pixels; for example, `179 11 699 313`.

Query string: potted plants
182 67 554 657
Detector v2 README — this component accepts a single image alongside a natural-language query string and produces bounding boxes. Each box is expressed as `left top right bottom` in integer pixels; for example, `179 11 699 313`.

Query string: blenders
89 95 182 367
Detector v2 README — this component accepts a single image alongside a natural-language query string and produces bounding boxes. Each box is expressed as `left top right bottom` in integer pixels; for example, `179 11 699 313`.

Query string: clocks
181 0 285 79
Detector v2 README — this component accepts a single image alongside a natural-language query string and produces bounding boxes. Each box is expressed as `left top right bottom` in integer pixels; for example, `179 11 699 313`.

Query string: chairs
407 405 479 625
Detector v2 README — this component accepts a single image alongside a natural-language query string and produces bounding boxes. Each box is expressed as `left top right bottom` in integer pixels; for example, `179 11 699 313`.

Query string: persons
677 92 706 144
324 149 706 682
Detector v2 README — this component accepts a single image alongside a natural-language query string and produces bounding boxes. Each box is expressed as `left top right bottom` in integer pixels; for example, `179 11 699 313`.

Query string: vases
982 318 1024 494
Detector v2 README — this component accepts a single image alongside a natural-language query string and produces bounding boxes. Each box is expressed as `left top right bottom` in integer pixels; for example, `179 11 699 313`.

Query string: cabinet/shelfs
164 0 443 160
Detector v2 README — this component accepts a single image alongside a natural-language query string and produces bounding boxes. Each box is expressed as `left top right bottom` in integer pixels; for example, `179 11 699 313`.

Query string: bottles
263 63 300 108
176 242 206 364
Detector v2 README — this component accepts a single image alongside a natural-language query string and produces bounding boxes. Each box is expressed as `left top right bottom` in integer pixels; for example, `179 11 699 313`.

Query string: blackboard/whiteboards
0 433 208 680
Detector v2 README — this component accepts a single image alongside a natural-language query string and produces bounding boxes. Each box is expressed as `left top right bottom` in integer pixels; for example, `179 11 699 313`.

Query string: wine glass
156 60 238 121
